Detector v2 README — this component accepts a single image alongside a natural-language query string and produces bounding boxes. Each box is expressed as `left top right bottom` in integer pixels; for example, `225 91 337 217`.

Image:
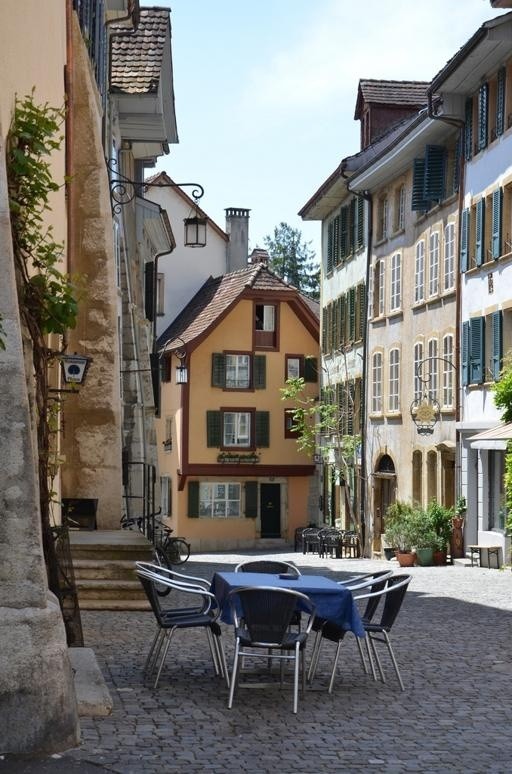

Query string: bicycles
120 506 191 597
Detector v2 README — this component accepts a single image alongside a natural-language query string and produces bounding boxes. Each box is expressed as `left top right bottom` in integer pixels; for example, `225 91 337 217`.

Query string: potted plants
382 496 469 566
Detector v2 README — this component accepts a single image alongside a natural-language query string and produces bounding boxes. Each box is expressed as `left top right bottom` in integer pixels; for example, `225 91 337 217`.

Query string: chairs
133 561 232 690
295 527 362 559
234 560 302 668
227 586 318 712
306 569 414 695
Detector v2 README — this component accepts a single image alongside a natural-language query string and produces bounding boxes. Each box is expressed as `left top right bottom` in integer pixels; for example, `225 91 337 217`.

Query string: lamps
149 336 189 384
47 350 94 393
105 155 207 248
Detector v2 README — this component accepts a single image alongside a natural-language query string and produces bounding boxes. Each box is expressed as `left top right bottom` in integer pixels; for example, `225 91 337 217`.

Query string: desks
205 572 366 692
467 546 502 569
